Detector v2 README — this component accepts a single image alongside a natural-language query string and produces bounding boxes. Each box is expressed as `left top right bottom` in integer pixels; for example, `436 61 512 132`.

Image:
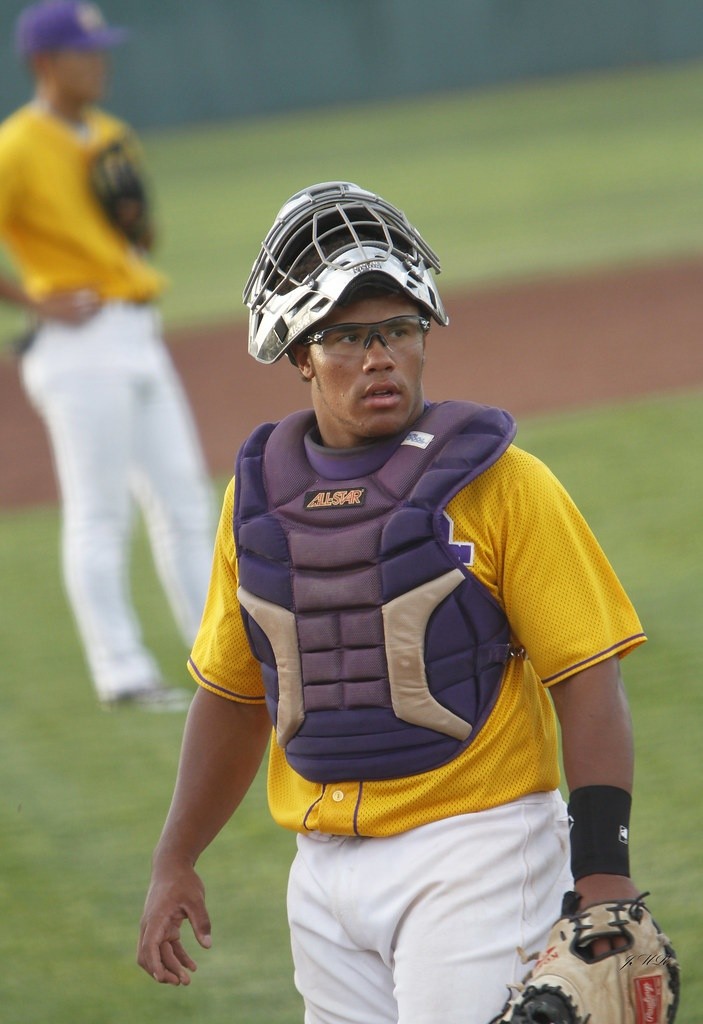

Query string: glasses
302 315 430 353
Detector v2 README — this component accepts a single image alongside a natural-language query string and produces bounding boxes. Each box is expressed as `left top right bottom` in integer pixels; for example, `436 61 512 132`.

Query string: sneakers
102 686 193 712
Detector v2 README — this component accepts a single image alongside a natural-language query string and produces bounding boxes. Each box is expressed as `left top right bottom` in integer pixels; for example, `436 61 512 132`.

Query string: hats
16 1 129 53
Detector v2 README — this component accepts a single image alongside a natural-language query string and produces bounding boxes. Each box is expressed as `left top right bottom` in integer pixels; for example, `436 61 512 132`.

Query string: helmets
241 182 450 362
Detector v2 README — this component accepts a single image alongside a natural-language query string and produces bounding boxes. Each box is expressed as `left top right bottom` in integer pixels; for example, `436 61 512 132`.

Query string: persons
137 182 681 1024
0 0 223 712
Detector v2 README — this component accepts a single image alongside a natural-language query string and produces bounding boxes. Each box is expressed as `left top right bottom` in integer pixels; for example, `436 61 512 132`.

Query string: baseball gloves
91 141 158 250
490 898 681 1023
